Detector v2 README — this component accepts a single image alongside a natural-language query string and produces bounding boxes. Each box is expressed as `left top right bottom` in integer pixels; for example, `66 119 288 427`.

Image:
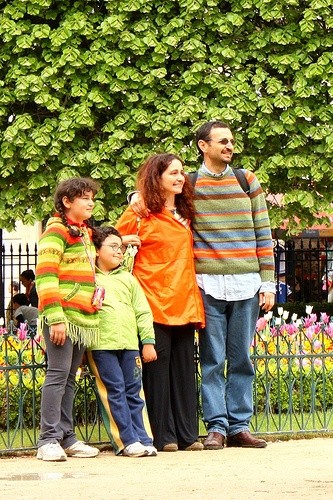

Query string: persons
12 293 38 338
35 177 142 461
87 226 157 457
6 281 19 327
115 153 205 451
127 122 275 450
21 270 38 308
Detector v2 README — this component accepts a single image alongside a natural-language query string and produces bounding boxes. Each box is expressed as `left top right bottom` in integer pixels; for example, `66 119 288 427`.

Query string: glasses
205 136 236 146
100 245 123 251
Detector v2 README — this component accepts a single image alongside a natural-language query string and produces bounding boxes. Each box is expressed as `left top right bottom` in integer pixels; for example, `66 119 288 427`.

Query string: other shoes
186 442 203 450
227 432 267 447
204 431 225 449
163 443 178 451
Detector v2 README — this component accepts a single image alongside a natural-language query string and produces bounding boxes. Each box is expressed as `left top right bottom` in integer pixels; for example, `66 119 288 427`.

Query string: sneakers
37 442 67 461
63 441 101 457
145 445 157 456
122 441 149 457
